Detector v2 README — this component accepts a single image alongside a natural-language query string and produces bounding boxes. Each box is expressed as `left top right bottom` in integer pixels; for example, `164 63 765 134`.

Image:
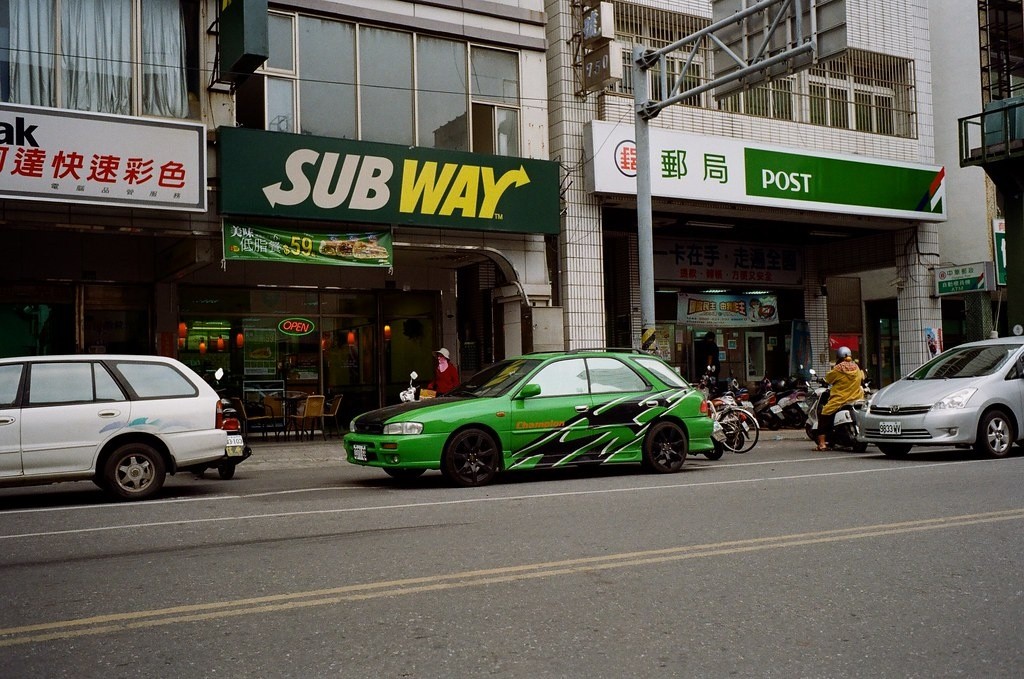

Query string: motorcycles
203 367 253 480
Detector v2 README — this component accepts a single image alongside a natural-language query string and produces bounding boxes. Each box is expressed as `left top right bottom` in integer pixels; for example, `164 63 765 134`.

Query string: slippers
812 446 826 451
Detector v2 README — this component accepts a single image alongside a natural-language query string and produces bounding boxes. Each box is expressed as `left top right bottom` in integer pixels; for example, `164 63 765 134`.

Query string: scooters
685 365 872 452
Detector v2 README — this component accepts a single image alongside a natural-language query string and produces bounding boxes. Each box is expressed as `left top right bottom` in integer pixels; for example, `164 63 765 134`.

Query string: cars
344 346 715 489
857 335 1023 458
0 355 227 502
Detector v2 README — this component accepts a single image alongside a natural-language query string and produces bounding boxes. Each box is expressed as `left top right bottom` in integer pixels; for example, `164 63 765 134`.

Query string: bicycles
715 405 761 455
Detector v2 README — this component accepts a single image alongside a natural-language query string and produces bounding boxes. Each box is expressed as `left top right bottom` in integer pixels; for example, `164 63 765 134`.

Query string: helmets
837 346 852 358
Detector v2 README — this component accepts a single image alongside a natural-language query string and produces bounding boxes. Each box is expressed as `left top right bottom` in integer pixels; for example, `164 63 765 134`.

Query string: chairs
288 395 327 443
231 397 286 442
311 394 343 442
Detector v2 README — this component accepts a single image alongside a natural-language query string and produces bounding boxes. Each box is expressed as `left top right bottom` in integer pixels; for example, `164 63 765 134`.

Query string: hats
432 348 452 361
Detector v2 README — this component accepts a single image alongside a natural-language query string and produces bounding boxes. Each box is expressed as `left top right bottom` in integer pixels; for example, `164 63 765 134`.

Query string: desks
274 397 307 440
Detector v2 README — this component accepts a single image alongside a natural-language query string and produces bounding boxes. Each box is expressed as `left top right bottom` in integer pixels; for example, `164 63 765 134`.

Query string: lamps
236 331 244 348
218 333 224 352
384 324 392 341
199 336 206 356
347 330 355 346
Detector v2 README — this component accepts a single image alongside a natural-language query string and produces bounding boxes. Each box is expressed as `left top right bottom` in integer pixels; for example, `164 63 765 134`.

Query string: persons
702 332 721 395
812 347 863 451
428 348 459 398
